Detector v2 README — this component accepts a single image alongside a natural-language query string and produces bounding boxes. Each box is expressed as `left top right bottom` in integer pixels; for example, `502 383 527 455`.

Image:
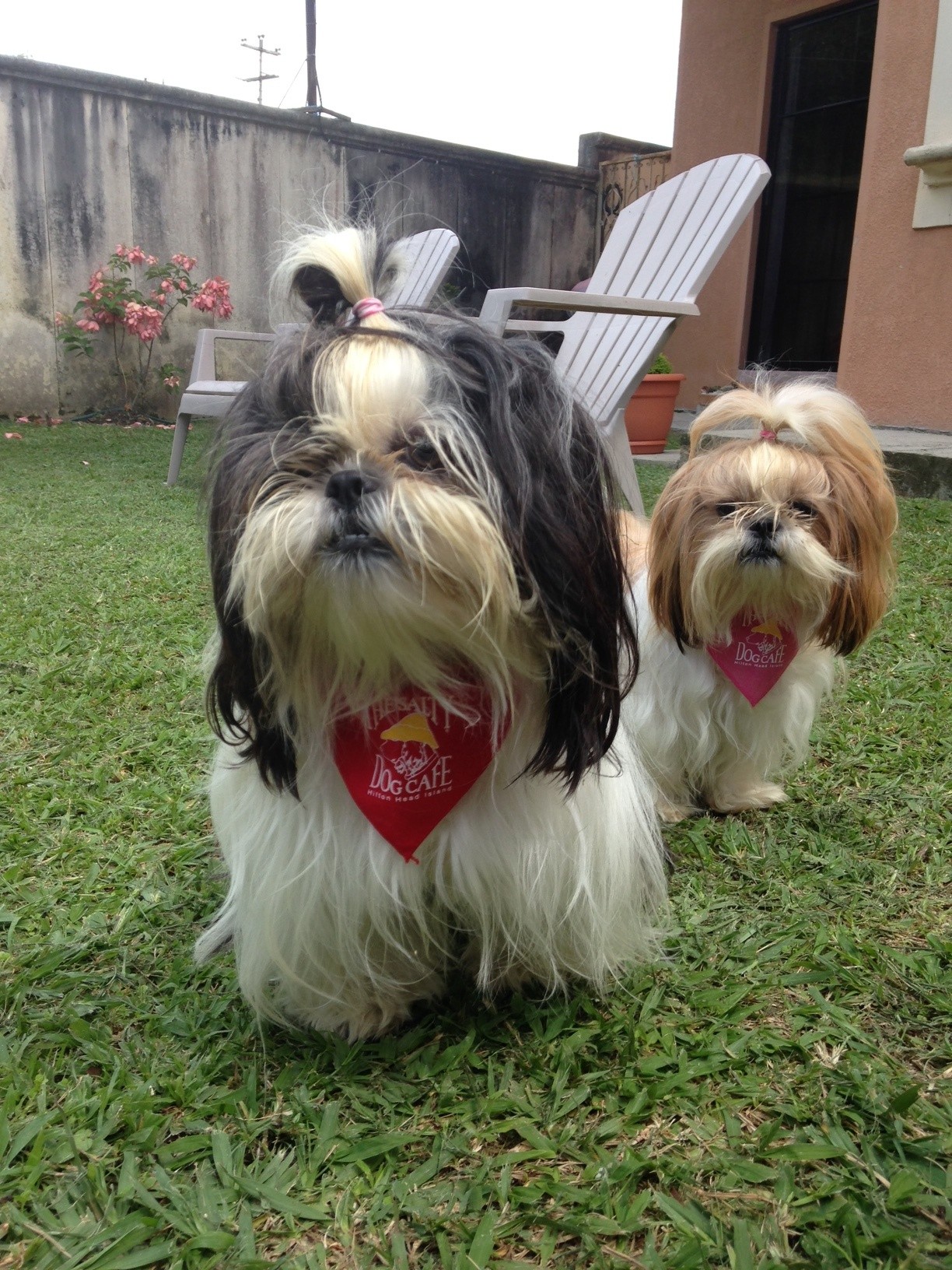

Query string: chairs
166 228 460 486
472 152 772 523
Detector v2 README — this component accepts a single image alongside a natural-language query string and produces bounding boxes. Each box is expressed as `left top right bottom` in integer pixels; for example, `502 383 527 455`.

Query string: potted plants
623 350 686 456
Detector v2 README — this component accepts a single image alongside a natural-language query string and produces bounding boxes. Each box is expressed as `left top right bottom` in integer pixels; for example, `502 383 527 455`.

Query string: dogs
196 227 676 1046
613 362 898 829
